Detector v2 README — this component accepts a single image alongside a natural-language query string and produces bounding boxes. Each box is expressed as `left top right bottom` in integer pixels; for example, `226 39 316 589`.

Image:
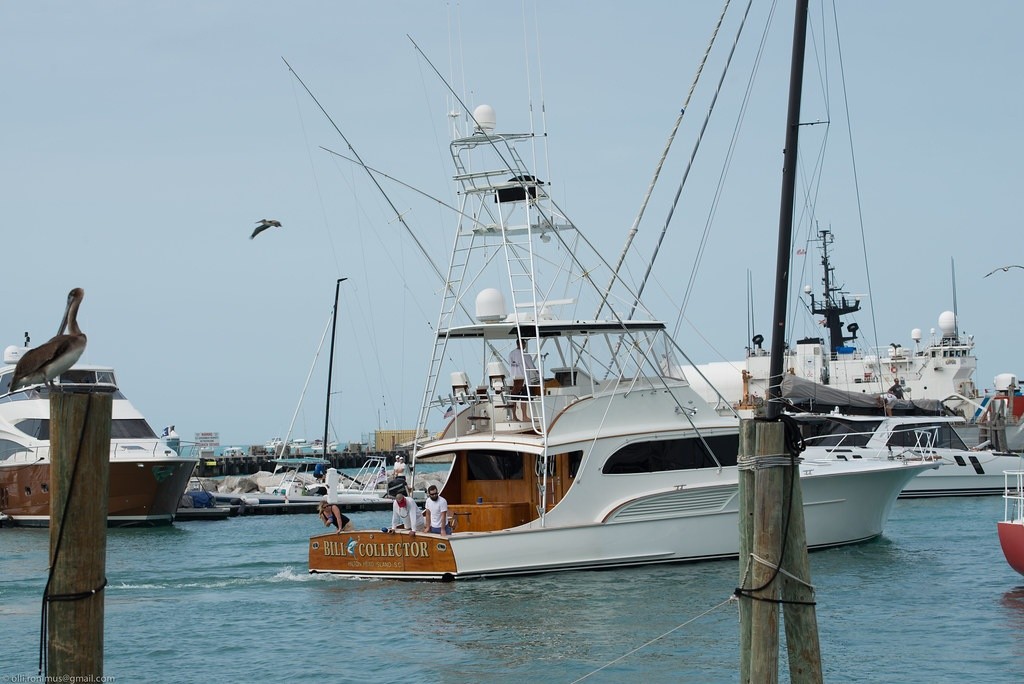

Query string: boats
715 219 1024 451
0 332 201 528
279 33 944 581
800 413 1024 499
997 467 1024 576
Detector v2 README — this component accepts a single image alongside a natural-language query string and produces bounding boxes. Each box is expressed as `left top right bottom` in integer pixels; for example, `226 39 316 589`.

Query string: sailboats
186 276 431 513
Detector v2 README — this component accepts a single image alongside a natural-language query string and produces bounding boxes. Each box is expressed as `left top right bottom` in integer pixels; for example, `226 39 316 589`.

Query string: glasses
431 493 439 496
401 459 403 460
323 505 328 509
397 458 399 459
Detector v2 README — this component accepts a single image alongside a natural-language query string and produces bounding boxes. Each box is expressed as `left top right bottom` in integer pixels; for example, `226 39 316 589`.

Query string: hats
396 455 400 458
400 457 404 459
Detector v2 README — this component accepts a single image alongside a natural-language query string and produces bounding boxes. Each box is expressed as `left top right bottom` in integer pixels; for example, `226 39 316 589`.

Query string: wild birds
7 286 89 394
984 264 1024 280
249 218 283 240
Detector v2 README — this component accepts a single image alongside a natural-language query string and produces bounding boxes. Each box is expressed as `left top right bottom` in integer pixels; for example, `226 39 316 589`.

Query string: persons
424 485 452 536
508 339 539 422
317 500 352 534
888 378 905 400
392 493 425 536
393 455 406 483
313 461 327 482
